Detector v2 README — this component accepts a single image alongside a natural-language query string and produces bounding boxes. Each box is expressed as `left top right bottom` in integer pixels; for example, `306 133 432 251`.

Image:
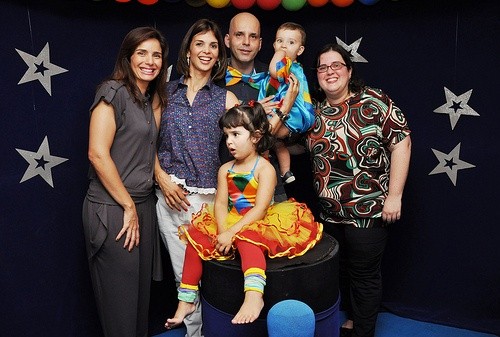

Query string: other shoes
339 327 353 337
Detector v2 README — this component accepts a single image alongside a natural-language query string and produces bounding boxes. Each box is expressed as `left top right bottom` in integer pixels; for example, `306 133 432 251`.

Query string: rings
178 200 182 205
293 82 298 86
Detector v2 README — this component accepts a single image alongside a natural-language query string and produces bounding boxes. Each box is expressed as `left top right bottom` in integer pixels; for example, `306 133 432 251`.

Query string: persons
215 9 301 201
151 17 299 337
162 99 278 332
260 21 316 186
287 42 413 337
79 25 174 337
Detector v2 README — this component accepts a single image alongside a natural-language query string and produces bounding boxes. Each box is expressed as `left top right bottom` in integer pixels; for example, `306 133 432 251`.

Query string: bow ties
224 65 265 90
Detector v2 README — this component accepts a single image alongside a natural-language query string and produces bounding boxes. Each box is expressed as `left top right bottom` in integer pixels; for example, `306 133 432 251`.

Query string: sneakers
280 172 295 185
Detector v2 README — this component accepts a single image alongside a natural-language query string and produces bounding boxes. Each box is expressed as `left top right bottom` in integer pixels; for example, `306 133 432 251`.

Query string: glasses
316 61 349 73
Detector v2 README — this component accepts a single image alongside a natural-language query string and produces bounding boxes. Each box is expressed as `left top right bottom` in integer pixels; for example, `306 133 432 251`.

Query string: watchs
274 108 290 121
